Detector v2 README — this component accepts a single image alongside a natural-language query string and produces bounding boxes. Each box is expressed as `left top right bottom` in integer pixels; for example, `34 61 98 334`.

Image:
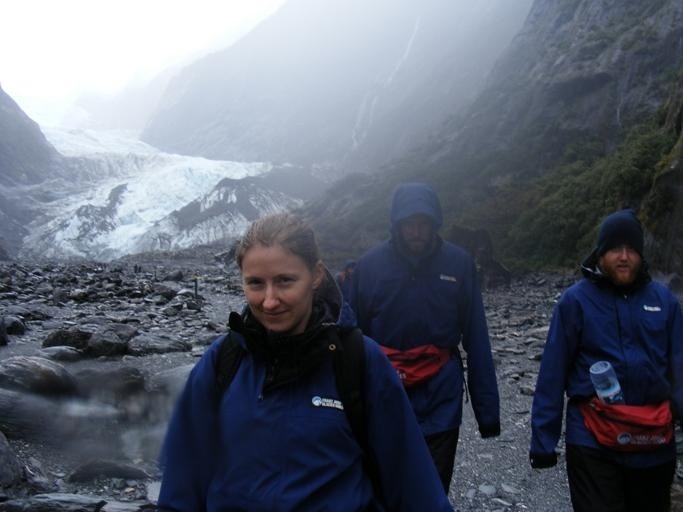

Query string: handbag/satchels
378 345 449 386
581 397 673 447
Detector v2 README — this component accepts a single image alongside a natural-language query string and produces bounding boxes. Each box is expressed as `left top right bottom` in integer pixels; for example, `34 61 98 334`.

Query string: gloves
479 427 500 438
529 451 558 468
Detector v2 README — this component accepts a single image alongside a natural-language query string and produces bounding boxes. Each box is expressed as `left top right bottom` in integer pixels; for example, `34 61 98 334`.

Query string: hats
595 208 643 258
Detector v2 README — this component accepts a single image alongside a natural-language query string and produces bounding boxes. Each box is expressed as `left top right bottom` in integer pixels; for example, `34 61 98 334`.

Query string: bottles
590 361 627 404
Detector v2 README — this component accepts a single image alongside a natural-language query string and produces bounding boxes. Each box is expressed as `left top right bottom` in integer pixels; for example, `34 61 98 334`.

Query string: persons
347 182 501 496
153 212 455 512
334 260 358 303
528 207 683 512
